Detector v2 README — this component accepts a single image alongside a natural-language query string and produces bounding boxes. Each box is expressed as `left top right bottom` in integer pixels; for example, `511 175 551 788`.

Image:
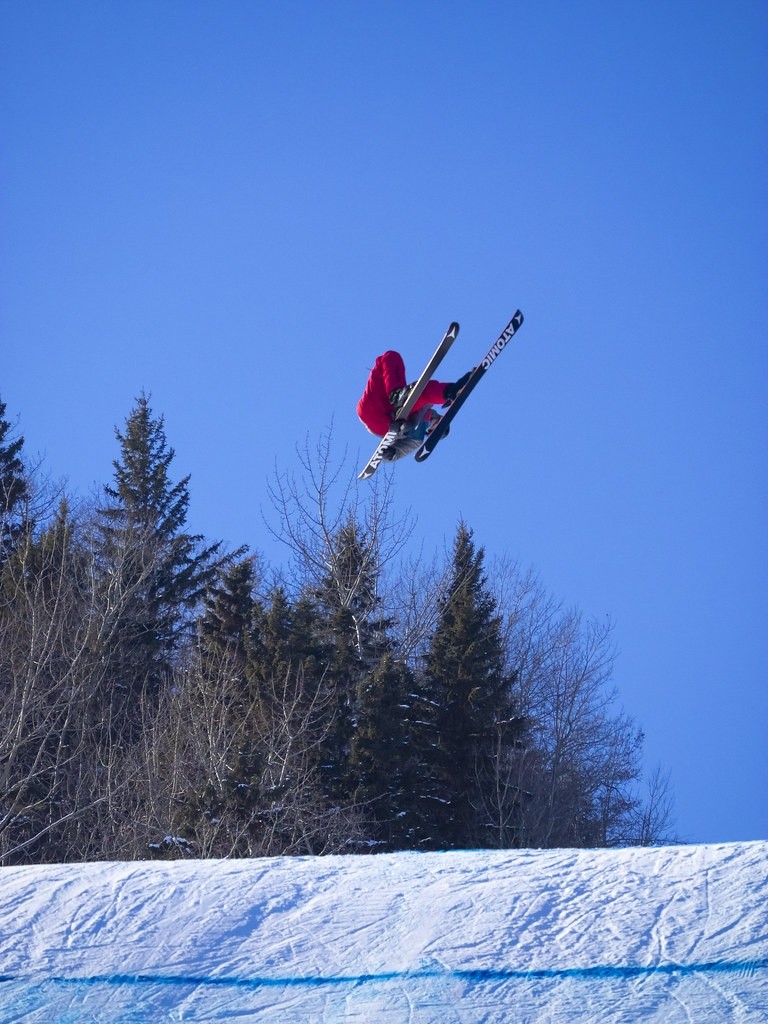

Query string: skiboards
354 307 525 483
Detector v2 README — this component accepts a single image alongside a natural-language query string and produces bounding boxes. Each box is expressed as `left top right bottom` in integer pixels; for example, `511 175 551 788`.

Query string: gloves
390 418 404 431
383 446 395 461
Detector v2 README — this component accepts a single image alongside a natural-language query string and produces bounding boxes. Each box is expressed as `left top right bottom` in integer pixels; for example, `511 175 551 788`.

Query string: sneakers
442 372 471 408
390 381 417 408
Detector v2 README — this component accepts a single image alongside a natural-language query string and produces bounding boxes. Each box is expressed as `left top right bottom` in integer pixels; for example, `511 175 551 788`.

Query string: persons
356 351 471 460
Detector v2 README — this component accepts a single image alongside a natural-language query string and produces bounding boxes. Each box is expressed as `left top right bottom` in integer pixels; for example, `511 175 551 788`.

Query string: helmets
424 415 450 438
